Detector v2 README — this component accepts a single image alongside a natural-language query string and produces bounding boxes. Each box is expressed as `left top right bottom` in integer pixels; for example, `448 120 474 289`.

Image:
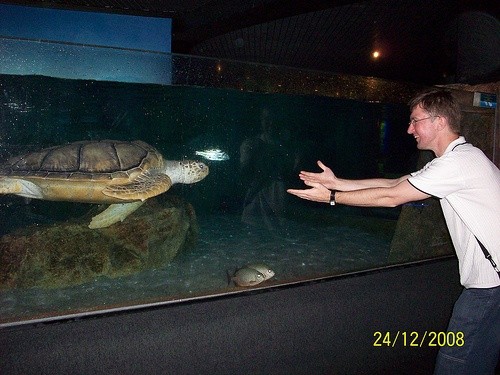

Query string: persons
286 87 500 374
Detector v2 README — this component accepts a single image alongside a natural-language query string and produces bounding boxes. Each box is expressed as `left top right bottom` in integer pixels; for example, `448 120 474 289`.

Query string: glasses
407 116 442 125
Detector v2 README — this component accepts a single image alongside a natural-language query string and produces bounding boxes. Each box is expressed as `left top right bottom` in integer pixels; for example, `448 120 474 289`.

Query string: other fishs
193 146 232 162
226 268 264 288
246 263 275 280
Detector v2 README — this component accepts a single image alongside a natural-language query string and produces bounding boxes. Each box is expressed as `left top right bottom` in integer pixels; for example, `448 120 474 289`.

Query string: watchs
328 190 337 206
